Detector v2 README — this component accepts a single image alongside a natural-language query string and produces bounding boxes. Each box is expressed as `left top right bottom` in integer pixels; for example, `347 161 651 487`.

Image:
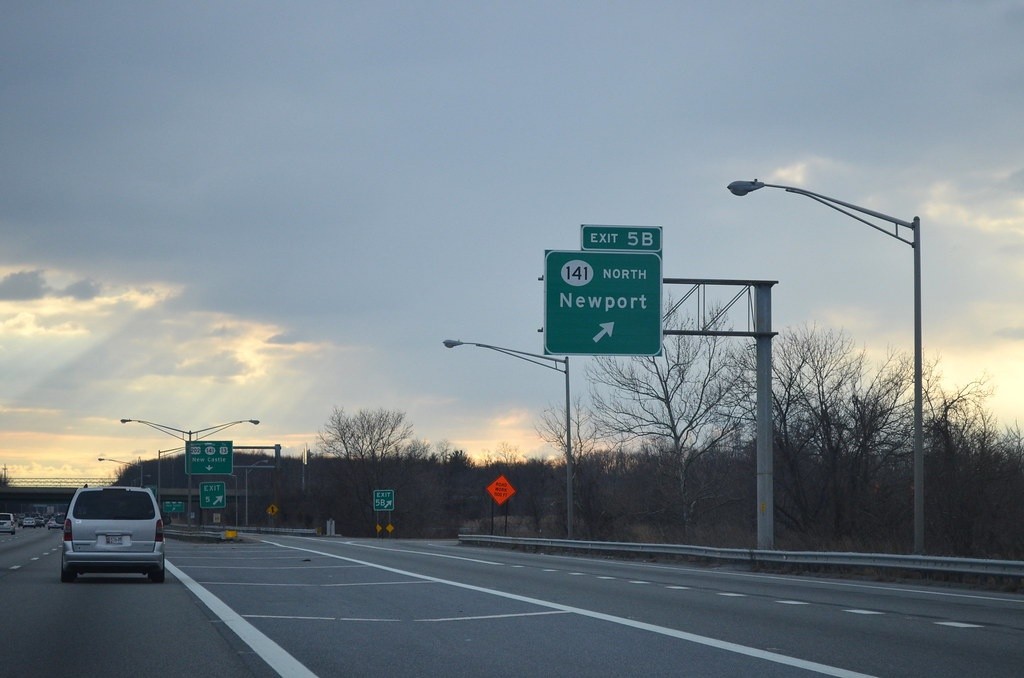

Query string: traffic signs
198 483 227 509
373 490 394 512
185 441 234 475
162 500 186 514
544 251 662 358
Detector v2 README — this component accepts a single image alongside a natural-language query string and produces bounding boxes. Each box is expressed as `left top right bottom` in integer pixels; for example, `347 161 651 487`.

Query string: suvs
55 484 171 583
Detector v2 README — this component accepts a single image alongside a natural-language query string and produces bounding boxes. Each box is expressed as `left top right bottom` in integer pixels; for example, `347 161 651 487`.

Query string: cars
0 513 63 534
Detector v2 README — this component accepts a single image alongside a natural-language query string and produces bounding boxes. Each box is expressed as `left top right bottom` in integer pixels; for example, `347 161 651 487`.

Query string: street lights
727 177 923 554
120 419 259 532
98 458 152 487
442 339 574 540
246 459 268 527
